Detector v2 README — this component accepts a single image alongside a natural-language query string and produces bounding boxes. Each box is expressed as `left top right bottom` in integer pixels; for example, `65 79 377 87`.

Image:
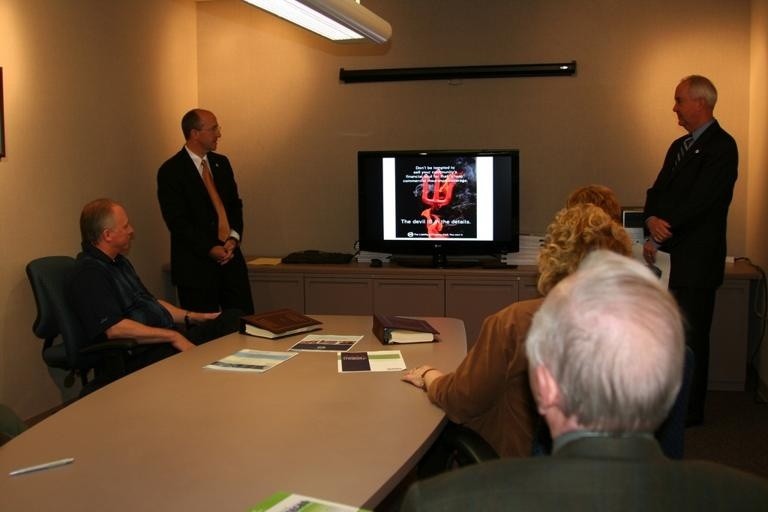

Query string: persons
565 184 622 226
399 247 768 512
401 201 632 462
641 73 739 446
66 198 222 387
157 108 255 343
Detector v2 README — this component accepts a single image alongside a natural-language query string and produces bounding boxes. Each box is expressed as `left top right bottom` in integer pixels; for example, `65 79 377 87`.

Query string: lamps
247 0 393 45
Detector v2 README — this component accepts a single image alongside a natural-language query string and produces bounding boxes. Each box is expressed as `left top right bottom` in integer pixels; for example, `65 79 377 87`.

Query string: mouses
371 259 383 267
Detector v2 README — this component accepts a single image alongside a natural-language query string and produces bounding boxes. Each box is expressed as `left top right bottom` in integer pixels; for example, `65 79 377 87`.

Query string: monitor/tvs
357 149 520 267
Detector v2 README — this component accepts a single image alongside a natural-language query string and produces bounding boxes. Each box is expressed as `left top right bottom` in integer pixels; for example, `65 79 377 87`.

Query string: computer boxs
621 207 644 244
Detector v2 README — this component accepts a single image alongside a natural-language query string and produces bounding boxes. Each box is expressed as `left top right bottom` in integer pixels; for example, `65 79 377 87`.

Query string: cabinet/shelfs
519 277 543 304
708 280 747 391
445 276 520 354
304 275 445 316
246 273 304 314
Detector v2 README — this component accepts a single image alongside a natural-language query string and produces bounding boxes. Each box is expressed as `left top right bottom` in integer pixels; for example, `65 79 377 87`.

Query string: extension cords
725 256 735 263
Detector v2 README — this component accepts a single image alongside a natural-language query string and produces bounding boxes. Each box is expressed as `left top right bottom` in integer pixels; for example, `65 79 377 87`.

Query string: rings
647 254 651 257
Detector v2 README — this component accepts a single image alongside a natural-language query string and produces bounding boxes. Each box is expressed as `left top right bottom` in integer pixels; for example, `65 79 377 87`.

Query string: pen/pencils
9 457 75 479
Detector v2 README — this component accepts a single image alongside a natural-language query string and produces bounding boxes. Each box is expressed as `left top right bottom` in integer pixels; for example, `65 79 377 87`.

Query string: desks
0 314 468 512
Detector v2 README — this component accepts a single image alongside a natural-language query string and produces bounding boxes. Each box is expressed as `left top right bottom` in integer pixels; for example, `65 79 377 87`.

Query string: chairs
25 256 142 395
452 344 695 464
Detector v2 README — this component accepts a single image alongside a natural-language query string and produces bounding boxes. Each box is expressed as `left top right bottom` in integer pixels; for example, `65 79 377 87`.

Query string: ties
201 160 230 243
673 134 693 170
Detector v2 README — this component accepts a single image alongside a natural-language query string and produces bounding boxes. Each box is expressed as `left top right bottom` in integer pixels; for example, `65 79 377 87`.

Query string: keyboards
281 252 352 264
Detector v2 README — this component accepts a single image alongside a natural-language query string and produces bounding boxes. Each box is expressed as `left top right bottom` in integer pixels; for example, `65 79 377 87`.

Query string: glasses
196 125 222 135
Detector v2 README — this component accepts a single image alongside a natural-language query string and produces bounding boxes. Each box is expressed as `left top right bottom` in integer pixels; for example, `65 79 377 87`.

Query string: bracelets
420 368 437 392
185 311 192 326
